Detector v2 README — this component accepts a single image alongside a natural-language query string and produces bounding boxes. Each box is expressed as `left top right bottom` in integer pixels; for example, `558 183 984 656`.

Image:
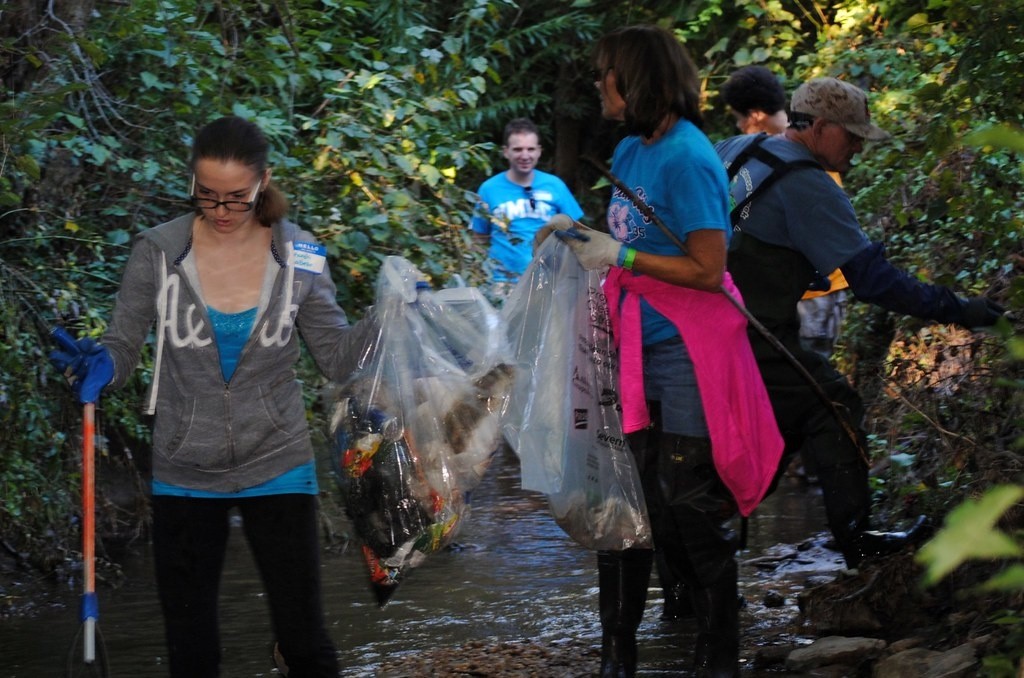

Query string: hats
790 77 891 140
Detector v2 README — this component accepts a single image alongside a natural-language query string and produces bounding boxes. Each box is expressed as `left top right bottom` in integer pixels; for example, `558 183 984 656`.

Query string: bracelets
616 244 637 268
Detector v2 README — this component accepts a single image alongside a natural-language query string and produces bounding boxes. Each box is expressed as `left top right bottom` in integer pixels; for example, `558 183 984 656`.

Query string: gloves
964 297 1006 327
48 328 114 403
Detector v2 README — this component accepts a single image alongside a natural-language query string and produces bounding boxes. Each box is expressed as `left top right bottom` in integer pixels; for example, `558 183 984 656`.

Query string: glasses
590 66 613 82
190 173 261 212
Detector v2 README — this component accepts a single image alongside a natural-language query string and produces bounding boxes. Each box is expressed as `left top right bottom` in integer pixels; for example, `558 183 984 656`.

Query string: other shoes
660 588 747 622
845 516 930 570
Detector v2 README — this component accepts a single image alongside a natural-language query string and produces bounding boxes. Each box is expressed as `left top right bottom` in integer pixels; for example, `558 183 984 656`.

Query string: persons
47 116 436 678
533 26 1007 678
466 115 586 311
